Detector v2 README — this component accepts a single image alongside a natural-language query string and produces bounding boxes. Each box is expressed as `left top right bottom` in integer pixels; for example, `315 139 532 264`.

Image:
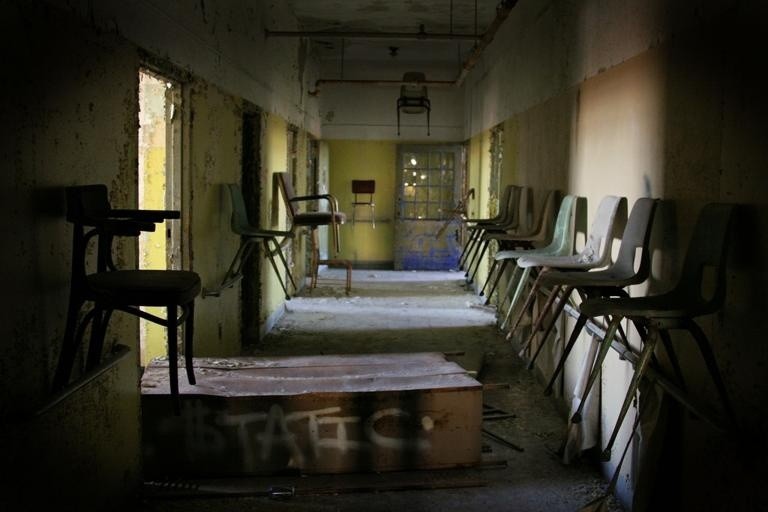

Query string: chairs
306 224 355 297
565 201 747 462
274 170 348 259
397 71 431 137
218 180 302 305
58 181 206 405
352 177 375 223
457 186 666 398
